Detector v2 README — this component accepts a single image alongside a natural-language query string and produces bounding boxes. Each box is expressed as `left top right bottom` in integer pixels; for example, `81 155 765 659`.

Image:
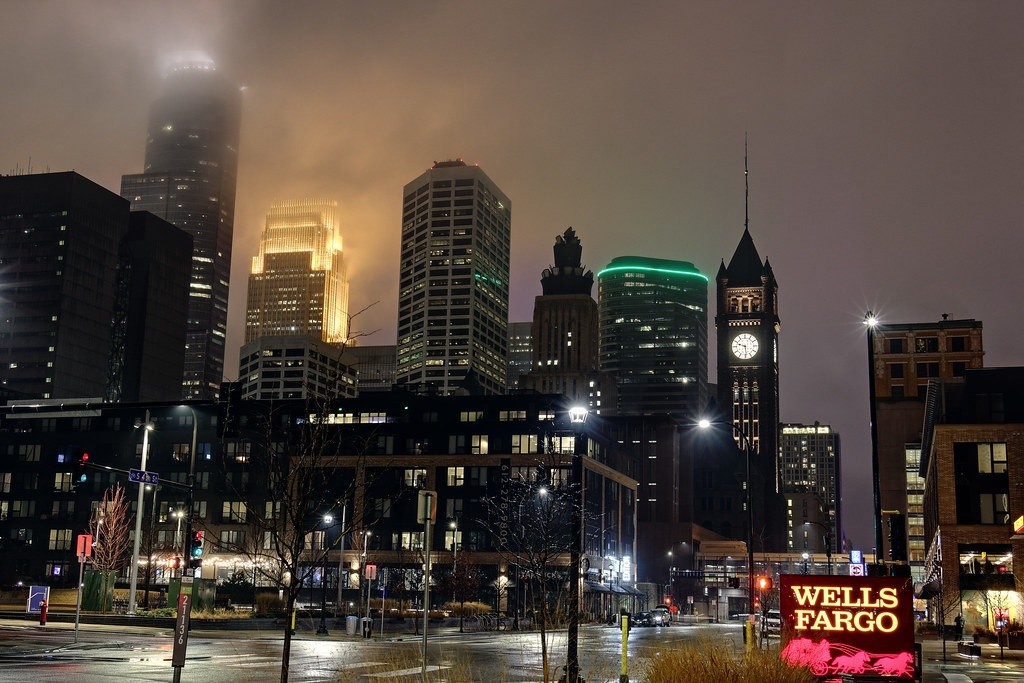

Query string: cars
759 609 780 638
631 604 673 627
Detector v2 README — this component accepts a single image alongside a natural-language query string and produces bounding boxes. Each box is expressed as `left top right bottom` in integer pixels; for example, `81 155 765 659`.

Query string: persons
971 558 995 574
954 612 966 641
916 613 921 626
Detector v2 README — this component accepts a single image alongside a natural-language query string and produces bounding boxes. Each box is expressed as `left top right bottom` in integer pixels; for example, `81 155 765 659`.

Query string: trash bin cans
361 617 373 636
346 616 358 637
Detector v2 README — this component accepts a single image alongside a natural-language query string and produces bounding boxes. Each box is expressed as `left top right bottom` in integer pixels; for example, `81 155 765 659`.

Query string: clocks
731 333 759 360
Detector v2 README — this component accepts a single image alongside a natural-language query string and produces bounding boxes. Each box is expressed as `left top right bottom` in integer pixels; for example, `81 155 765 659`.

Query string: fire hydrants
38 600 48 625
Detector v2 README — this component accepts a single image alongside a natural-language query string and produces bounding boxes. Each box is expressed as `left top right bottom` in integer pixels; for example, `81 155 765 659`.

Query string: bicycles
473 612 506 631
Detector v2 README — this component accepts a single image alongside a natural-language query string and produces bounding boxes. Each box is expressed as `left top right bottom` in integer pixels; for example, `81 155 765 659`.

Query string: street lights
361 530 373 607
698 420 755 643
315 515 332 636
608 564 614 626
671 541 686 603
172 510 183 578
803 521 831 575
450 515 457 603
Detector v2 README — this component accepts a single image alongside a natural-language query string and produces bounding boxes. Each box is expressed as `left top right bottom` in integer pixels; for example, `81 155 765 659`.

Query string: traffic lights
191 531 203 557
77 452 90 483
756 576 769 589
664 598 672 604
